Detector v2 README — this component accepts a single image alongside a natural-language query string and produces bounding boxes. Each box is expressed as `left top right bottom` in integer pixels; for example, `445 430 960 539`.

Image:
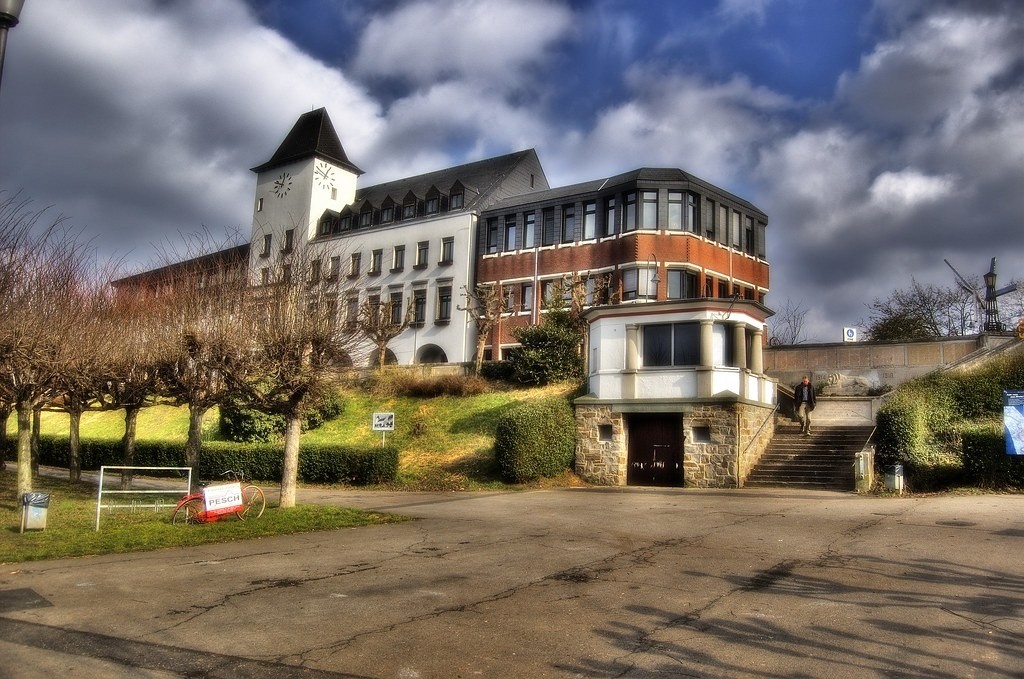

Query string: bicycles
172 469 265 525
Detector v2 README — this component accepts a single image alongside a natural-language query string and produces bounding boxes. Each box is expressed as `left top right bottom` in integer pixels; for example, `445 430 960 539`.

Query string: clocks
314 162 336 189
274 172 293 198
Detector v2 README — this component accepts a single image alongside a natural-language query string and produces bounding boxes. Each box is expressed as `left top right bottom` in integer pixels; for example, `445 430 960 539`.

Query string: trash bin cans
883 462 904 490
20 490 50 532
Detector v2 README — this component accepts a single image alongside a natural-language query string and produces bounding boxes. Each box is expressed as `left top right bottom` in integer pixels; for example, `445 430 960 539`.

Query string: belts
802 401 807 402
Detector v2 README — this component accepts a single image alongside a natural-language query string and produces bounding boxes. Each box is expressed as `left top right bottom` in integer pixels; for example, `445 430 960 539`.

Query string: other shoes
806 432 810 435
799 430 804 432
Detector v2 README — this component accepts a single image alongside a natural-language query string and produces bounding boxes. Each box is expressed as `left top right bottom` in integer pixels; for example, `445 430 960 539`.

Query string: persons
794 375 817 435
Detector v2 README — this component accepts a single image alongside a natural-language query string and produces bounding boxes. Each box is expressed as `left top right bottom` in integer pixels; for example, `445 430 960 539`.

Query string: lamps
646 253 661 302
